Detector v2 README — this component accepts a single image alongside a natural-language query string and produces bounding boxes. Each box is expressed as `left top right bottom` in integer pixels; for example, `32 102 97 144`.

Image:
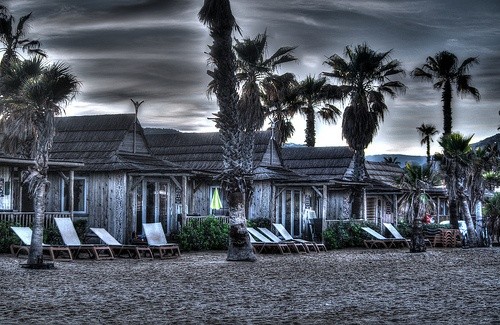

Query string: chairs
258 227 317 254
10 226 73 261
143 222 182 260
362 226 405 249
246 227 307 254
248 234 292 254
424 220 462 248
54 218 114 260
383 222 432 248
274 223 328 253
90 227 154 259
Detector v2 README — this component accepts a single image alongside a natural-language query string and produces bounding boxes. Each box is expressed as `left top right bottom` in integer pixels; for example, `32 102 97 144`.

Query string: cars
439 220 478 246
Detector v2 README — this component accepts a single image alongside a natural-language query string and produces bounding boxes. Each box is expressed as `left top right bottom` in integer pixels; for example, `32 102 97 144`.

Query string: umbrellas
210 187 223 217
